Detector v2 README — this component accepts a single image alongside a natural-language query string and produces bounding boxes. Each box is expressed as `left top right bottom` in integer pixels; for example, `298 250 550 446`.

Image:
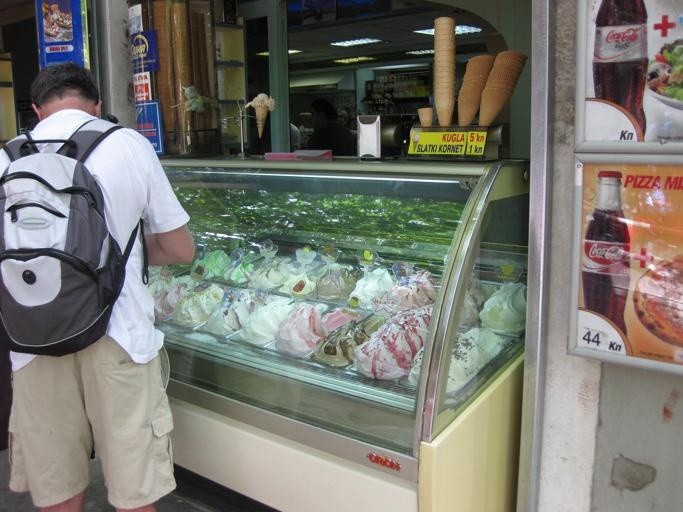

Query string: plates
576 307 631 356
584 97 645 143
645 85 683 110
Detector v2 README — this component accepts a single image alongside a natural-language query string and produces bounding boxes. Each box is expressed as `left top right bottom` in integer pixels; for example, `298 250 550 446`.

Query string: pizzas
633 253 682 351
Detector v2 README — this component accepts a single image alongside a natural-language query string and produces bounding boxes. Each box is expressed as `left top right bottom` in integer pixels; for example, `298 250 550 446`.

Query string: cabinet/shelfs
124 148 528 512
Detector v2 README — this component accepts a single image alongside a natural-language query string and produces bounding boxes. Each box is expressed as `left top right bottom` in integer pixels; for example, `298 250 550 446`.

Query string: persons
0 64 195 511
335 105 351 126
308 97 353 155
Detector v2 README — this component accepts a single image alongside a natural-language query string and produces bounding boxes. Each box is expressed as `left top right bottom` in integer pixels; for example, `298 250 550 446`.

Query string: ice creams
251 93 276 140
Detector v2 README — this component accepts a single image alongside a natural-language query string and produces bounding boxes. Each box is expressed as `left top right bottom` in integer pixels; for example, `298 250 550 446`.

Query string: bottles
593 1 647 136
581 171 630 340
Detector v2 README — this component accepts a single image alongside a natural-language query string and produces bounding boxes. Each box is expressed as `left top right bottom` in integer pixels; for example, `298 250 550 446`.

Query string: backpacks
0 118 139 357
